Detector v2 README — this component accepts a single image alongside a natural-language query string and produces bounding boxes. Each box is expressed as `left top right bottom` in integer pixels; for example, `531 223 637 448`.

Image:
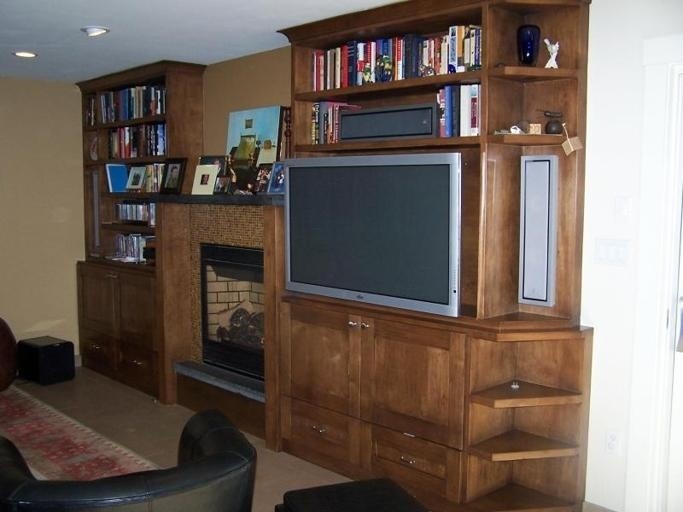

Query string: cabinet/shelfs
276 1 595 319
278 290 594 512
77 259 202 405
75 59 208 272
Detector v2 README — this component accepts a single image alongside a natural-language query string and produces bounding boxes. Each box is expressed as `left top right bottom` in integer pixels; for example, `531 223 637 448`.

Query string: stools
15 336 75 386
274 477 426 512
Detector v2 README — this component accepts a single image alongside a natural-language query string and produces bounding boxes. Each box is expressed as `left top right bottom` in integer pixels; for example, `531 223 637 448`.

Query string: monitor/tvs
284 153 460 320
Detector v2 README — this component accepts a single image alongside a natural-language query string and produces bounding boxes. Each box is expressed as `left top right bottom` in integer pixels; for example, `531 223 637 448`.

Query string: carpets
0 385 159 481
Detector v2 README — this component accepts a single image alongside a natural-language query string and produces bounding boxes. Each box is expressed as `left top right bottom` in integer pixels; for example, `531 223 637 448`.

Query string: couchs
0 317 256 511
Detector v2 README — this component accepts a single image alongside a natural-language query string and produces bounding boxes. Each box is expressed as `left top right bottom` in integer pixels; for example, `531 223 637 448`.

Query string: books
113 232 155 263
311 25 482 145
116 201 156 227
86 84 167 160
127 163 165 193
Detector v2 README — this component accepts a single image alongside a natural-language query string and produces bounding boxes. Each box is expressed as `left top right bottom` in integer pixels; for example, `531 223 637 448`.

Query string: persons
201 175 209 185
255 140 277 168
257 167 270 190
167 166 178 188
